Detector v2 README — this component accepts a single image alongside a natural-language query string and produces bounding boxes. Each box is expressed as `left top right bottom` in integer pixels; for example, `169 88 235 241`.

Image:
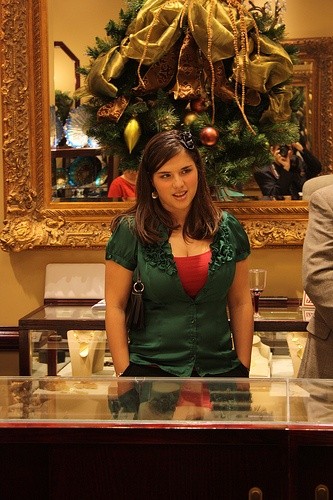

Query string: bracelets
116 372 124 377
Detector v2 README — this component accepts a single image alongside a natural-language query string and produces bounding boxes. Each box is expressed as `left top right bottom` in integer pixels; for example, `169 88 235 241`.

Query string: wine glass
248 269 268 318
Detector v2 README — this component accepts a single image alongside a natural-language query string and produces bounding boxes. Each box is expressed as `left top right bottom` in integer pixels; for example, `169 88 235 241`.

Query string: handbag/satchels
125 262 147 333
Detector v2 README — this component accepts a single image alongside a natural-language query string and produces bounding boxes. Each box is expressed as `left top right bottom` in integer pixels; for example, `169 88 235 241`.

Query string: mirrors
0 0 332 253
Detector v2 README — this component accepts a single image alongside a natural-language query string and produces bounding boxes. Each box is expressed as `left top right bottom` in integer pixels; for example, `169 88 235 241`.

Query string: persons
105 129 255 376
296 185 333 423
252 142 323 197
117 378 252 420
108 156 140 202
